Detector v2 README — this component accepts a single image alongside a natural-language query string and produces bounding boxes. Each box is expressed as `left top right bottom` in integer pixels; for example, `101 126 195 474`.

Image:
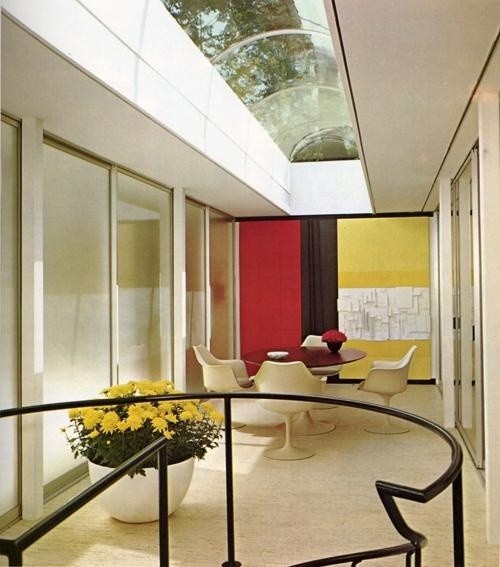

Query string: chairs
358 345 419 434
253 361 327 460
300 334 342 409
193 343 253 429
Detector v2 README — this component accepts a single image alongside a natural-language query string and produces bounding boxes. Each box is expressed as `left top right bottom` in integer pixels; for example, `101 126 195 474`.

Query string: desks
244 347 367 435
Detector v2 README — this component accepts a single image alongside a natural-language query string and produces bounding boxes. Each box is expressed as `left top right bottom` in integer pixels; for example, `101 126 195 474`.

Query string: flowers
59 379 247 478
321 329 347 343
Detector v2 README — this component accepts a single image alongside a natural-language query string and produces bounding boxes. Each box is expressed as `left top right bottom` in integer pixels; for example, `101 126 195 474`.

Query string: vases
87 456 194 523
327 343 342 352
